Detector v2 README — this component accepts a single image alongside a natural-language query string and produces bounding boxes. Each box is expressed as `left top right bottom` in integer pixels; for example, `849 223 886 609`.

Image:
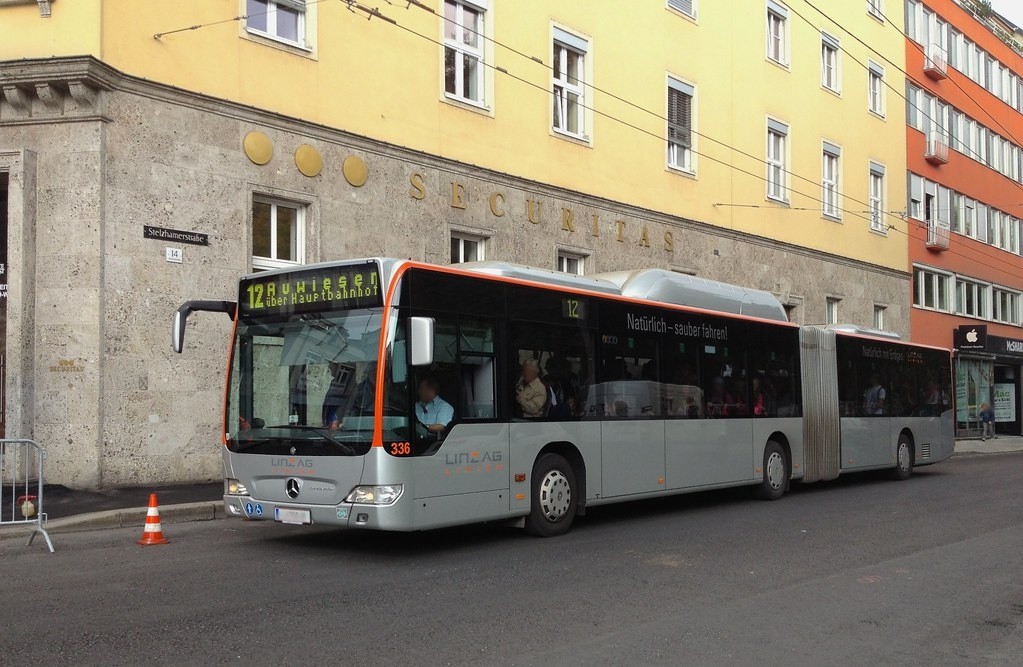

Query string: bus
172 256 958 537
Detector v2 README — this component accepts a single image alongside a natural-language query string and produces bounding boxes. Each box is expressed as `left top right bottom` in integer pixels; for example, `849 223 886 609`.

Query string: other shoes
990 438 994 441
982 437 985 441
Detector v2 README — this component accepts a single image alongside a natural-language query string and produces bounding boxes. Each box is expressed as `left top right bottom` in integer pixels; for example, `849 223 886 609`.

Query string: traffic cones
136 494 170 546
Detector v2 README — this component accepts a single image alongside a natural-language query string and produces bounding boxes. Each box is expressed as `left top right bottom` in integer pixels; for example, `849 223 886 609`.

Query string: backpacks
546 380 571 417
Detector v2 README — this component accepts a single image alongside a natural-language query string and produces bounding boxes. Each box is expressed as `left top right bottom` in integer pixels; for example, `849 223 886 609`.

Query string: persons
330 359 393 431
517 352 797 417
322 363 347 427
413 376 455 430
979 401 997 442
841 362 921 416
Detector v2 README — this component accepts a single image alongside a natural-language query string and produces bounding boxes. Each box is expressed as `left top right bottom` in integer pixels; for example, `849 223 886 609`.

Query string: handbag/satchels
515 385 524 417
